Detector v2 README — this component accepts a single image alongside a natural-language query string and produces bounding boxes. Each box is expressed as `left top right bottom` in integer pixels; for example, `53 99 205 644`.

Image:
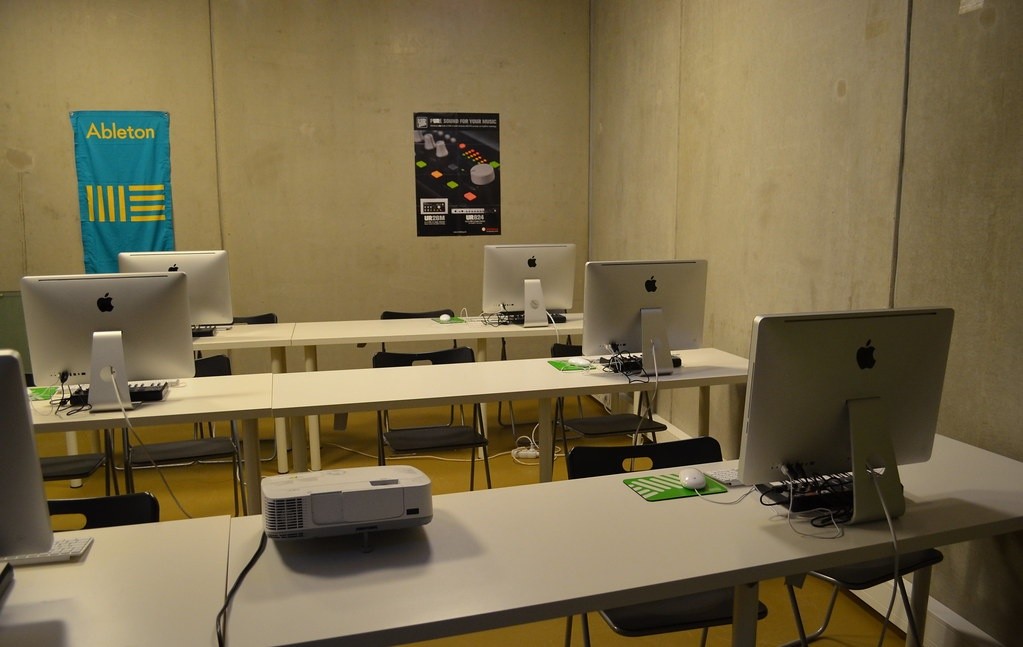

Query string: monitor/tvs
20 272 197 386
737 305 956 524
481 243 575 328
0 349 54 557
582 258 708 376
119 250 232 326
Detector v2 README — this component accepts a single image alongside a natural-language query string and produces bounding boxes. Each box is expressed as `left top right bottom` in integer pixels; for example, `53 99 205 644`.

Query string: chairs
38 313 948 647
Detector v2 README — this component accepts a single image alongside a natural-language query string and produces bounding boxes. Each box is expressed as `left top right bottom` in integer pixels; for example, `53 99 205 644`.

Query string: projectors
262 465 433 541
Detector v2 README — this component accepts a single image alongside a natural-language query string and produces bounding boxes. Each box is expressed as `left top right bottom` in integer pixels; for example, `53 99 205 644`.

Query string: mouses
569 357 591 367
440 313 451 320
679 467 706 490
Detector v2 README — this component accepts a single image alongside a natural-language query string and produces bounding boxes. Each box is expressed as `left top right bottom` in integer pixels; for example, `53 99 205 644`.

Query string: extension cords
511 449 538 459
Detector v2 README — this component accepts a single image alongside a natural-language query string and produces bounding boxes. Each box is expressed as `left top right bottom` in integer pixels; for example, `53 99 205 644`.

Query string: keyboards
0 537 94 566
703 468 741 485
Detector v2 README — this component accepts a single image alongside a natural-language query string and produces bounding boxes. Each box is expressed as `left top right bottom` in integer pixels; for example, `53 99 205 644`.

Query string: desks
272 349 752 518
0 514 224 646
29 374 273 525
293 311 585 474
191 322 292 473
232 458 1023 647
